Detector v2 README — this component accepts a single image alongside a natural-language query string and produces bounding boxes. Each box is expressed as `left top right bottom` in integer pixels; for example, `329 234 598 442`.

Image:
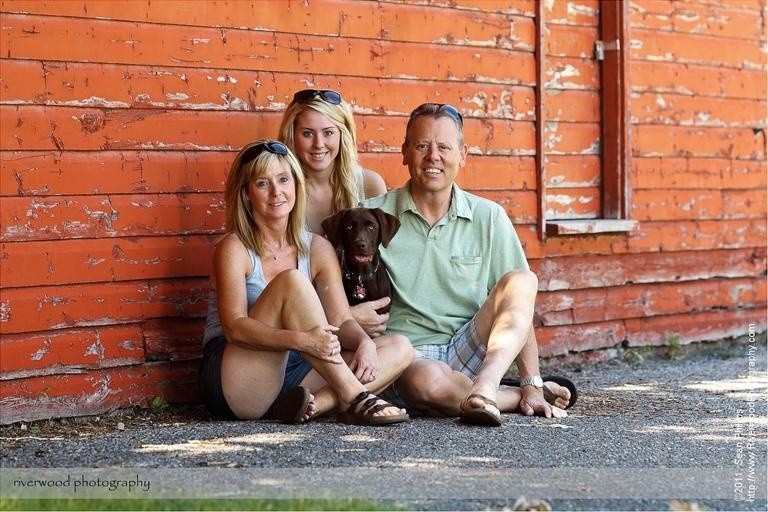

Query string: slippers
339 392 408 425
499 376 578 409
460 394 501 427
276 386 317 424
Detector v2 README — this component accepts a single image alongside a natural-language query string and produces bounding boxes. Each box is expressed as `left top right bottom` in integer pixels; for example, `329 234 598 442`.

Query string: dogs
320 207 401 340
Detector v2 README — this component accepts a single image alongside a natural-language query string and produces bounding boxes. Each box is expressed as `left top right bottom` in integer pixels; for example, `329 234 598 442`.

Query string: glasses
239 142 288 177
292 90 342 111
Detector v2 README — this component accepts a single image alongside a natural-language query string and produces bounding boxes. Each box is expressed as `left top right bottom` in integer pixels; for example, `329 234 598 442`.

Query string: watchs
519 374 545 390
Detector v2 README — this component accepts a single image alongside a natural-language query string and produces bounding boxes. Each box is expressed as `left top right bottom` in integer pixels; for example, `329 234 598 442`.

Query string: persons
363 102 578 429
278 84 386 234
198 137 416 425
